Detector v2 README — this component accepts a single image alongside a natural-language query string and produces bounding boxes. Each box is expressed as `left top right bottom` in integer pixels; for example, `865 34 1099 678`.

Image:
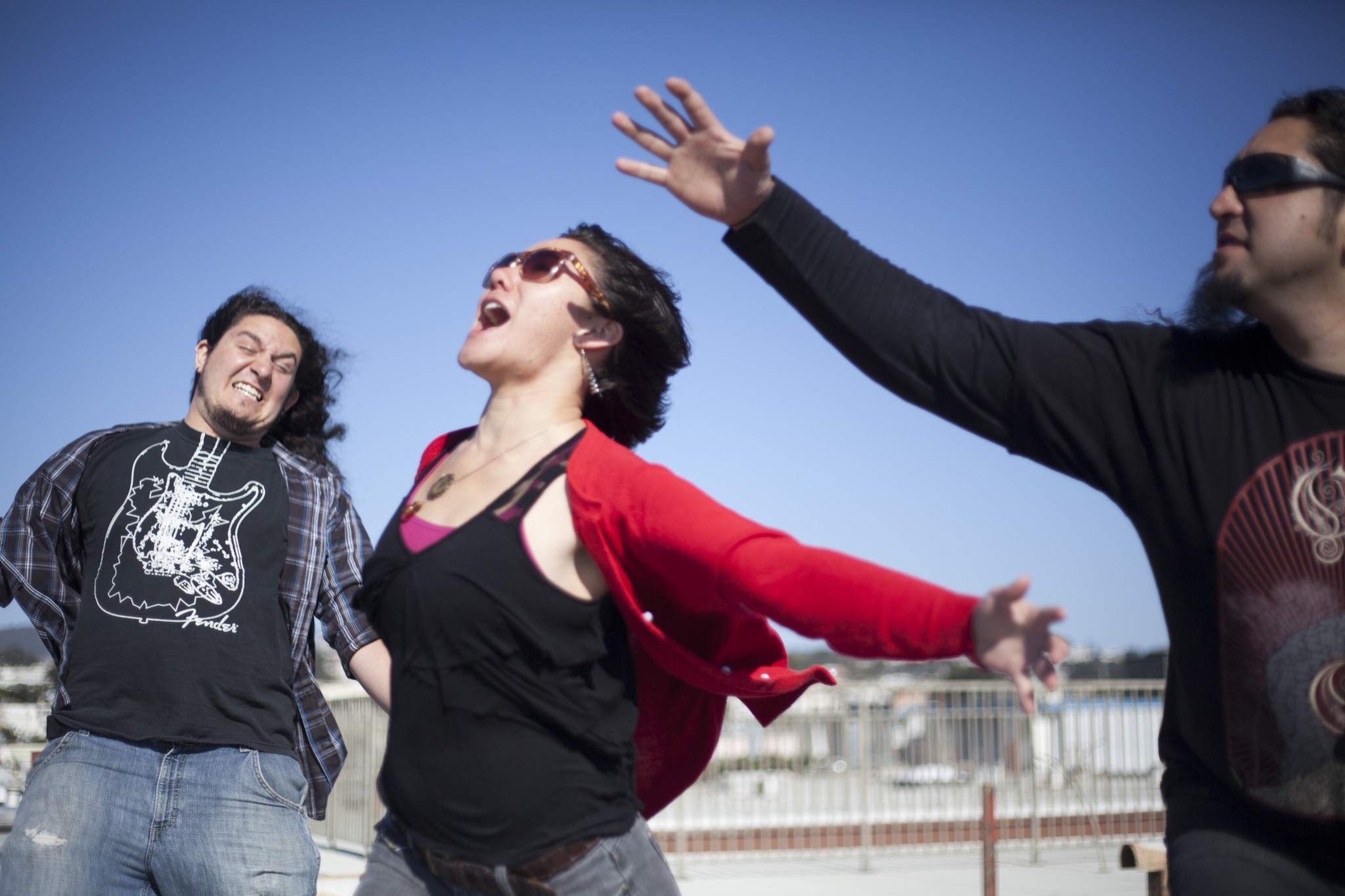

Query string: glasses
1222 151 1344 193
481 247 615 320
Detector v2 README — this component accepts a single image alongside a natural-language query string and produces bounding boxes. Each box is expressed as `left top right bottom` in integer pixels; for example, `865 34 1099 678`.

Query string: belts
373 813 600 896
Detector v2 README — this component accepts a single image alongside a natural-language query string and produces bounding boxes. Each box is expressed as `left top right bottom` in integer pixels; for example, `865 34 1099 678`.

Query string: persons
359 225 1069 896
0 284 395 896
613 71 1345 896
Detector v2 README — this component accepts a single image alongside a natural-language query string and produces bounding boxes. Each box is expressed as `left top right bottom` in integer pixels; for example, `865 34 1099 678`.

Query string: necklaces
427 408 586 501
401 439 476 526
